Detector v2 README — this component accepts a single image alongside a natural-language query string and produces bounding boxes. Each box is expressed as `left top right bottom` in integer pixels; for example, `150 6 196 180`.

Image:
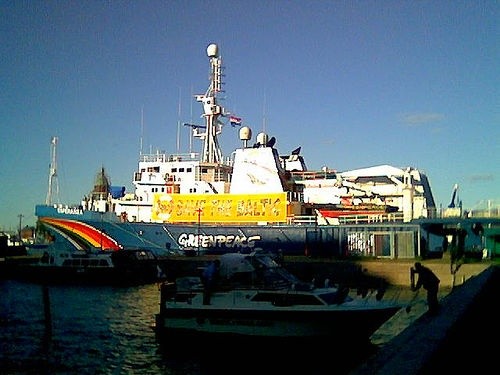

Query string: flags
229 115 242 128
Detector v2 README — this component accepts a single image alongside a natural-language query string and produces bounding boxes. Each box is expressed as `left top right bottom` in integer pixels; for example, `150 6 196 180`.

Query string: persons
199 259 220 305
443 237 448 252
420 236 426 257
411 261 442 312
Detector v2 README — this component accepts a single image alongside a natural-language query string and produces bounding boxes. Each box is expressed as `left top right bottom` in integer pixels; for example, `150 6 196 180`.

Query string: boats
159 240 427 339
0 44 449 287
